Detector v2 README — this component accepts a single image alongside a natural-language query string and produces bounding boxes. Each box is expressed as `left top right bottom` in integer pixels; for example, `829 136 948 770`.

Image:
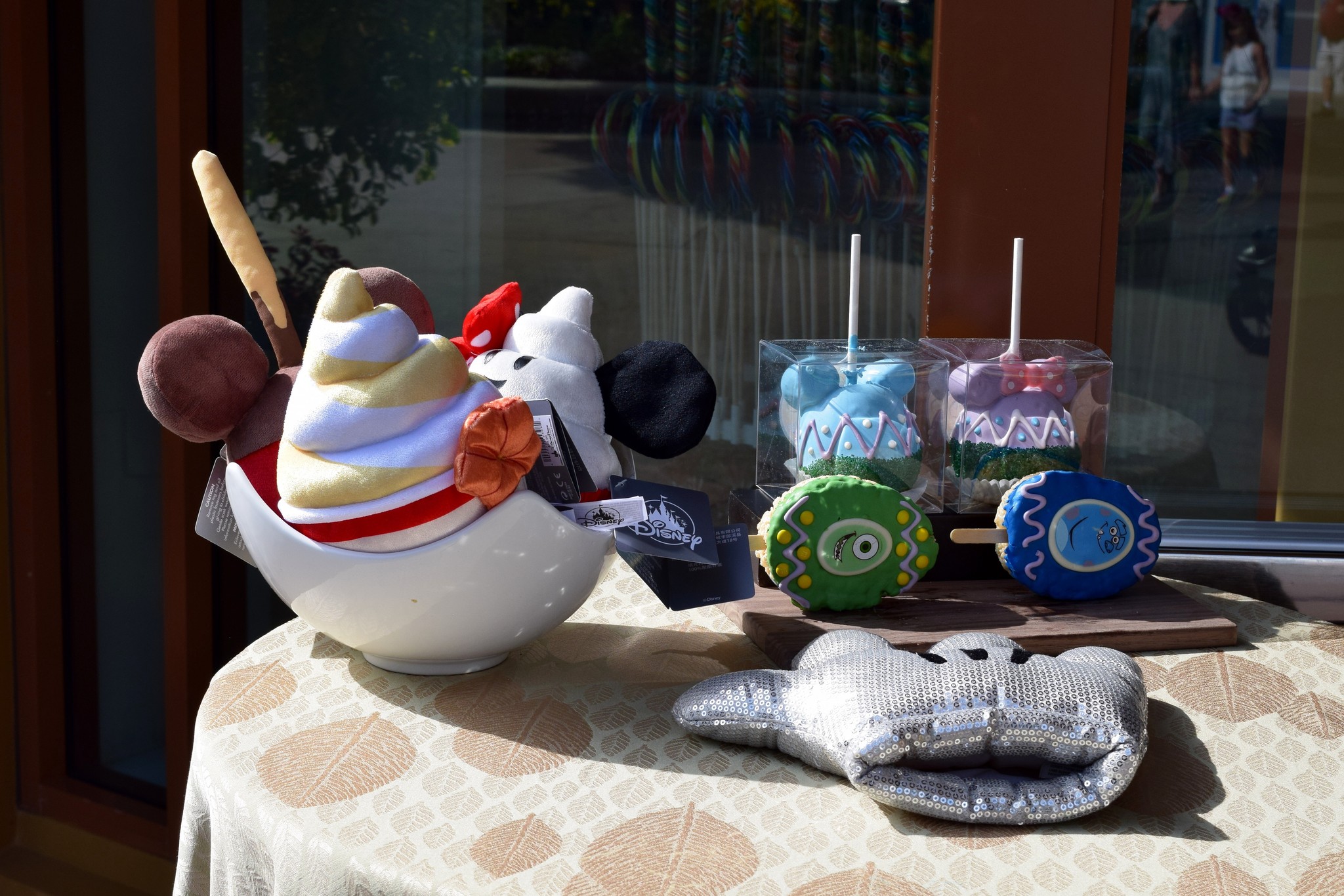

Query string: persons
1123 0 1203 222
1317 0 1344 115
1199 3 1272 204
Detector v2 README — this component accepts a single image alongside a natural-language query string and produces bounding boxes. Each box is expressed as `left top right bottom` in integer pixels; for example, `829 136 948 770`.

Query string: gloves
675 629 1150 825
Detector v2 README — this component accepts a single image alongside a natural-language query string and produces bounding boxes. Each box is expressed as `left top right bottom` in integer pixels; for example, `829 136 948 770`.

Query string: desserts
135 150 717 554
779 356 922 489
950 352 1080 503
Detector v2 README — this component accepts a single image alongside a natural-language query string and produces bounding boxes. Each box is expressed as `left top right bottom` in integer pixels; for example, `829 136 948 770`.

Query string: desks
168 550 1344 896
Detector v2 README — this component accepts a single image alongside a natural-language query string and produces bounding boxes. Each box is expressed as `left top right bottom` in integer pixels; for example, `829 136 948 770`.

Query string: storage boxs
755 339 950 514
916 339 1113 511
